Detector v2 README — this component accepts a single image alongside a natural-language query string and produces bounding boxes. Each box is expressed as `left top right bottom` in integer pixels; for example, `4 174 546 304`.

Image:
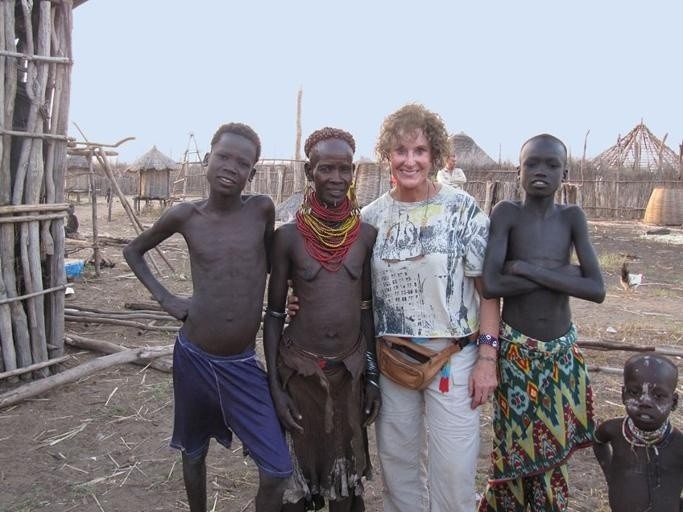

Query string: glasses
380 220 425 262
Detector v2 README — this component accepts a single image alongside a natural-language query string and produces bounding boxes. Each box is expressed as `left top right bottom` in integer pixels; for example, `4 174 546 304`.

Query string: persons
433 155 468 192
590 351 682 512
282 103 494 511
63 204 79 240
478 134 609 512
121 121 293 512
258 126 383 512
104 188 110 208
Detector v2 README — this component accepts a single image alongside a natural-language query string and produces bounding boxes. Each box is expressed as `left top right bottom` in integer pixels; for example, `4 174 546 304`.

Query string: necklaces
386 184 430 240
620 414 675 489
292 186 364 274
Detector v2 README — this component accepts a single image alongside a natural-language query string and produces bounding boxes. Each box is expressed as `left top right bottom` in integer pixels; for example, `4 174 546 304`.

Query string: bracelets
363 378 379 389
362 350 379 377
477 334 500 351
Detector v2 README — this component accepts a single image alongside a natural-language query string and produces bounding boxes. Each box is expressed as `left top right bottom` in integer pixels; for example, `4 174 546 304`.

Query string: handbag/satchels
373 336 458 390
